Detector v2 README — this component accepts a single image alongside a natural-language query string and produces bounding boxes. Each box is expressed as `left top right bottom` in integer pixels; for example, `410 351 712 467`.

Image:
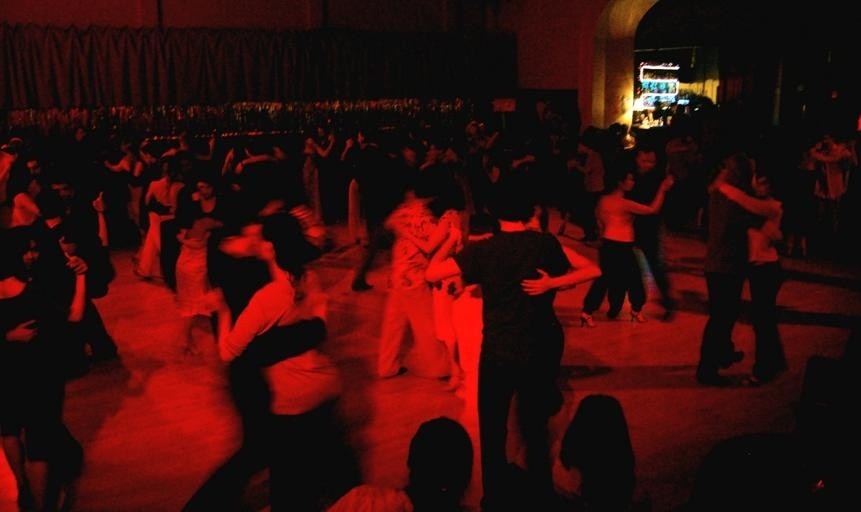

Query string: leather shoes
695 352 744 389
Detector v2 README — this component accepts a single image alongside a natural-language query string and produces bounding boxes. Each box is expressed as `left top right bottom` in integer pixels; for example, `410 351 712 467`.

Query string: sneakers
418 366 464 391
606 310 621 321
175 336 202 354
373 363 400 379
657 304 678 321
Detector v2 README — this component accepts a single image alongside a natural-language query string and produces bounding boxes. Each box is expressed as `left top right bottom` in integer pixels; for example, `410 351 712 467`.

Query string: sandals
742 370 772 389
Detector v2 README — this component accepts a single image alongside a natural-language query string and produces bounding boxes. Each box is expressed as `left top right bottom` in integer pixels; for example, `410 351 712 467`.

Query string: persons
0 92 859 511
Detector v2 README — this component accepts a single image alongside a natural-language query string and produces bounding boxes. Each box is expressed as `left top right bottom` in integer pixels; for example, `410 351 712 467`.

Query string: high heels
579 306 596 327
628 309 646 324
